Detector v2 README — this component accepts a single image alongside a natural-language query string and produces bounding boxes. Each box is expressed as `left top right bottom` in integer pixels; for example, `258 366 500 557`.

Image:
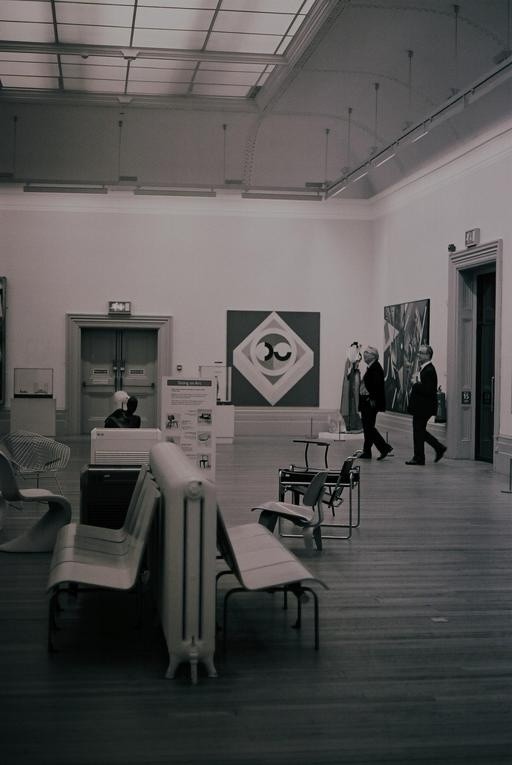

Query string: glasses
419 350 426 356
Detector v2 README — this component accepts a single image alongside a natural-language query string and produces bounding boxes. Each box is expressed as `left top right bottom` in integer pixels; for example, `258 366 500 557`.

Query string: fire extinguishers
433 385 446 422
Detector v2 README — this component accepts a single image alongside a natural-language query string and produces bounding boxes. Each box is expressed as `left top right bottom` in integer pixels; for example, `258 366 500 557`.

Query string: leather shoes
355 451 372 460
435 445 447 463
377 447 394 460
406 457 425 466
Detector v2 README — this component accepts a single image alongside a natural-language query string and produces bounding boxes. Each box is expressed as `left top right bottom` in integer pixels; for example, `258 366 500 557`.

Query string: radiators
148 441 219 681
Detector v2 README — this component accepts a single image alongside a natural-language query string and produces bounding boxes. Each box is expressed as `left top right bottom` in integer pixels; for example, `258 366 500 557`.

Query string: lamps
241 188 323 201
134 183 216 197
328 0 512 198
22 180 108 195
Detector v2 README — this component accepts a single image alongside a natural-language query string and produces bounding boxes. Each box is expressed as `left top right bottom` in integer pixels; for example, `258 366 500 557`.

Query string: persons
354 345 393 461
103 395 142 429
404 344 447 466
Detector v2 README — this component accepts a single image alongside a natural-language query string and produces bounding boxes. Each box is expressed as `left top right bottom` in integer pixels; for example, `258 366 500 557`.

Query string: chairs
250 438 361 559
215 503 331 653
0 430 71 496
43 461 162 653
0 449 72 554
